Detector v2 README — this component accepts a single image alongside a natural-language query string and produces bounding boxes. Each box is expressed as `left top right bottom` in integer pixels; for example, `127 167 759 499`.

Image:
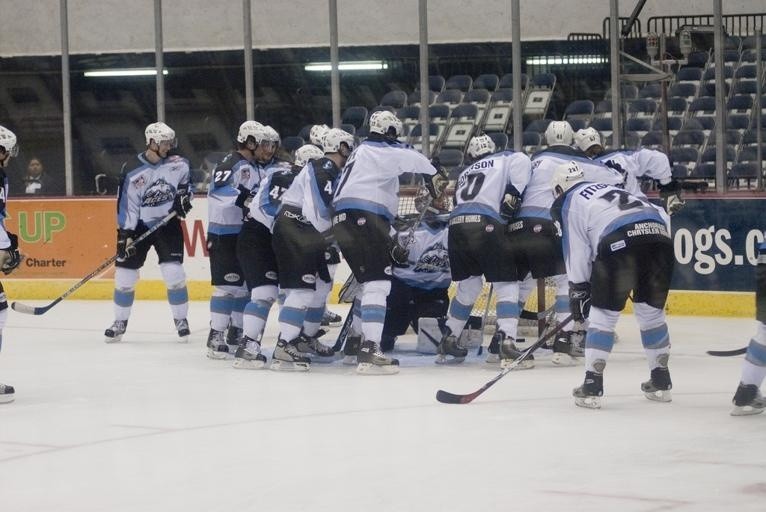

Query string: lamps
84 66 168 78
646 28 692 158
305 60 388 74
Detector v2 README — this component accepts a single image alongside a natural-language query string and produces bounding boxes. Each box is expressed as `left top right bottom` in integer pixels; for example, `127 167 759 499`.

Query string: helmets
466 132 496 158
545 119 574 147
308 123 330 144
264 124 281 143
294 144 324 168
550 161 589 197
144 121 176 159
319 127 356 156
368 110 403 137
0 127 18 162
415 184 438 213
573 126 603 152
236 119 271 152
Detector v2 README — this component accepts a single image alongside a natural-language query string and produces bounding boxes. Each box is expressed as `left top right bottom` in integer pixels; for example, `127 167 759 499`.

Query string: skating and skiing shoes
104 318 128 344
2 379 14 402
206 327 229 360
500 339 536 369
486 335 500 363
436 336 468 364
641 369 673 403
551 334 582 368
271 339 312 371
355 339 400 376
516 313 554 341
226 326 244 354
174 317 190 343
342 335 365 365
729 385 766 419
319 307 345 327
572 371 604 410
291 334 336 363
234 335 268 368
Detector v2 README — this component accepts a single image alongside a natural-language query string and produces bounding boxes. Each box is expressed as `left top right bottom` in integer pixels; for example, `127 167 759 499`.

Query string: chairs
594 101 622 115
471 74 499 92
414 74 445 94
396 106 421 142
728 35 742 50
407 122 440 157
667 97 687 118
734 79 758 94
737 50 758 64
640 131 673 151
281 137 305 155
624 118 651 134
725 95 754 110
652 117 682 134
481 89 514 133
704 66 733 81
701 145 736 164
726 113 749 130
562 100 594 131
499 72 530 101
739 146 766 162
523 131 541 156
626 97 657 119
713 49 741 66
444 75 472 93
670 129 706 150
341 106 368 130
524 119 553 151
753 113 766 127
437 103 479 153
341 124 356 136
743 127 766 144
735 64 757 80
668 147 699 178
299 124 314 145
606 129 640 153
621 85 638 101
677 66 703 82
693 164 717 179
640 84 663 101
356 127 370 142
590 117 613 132
729 162 758 188
200 151 228 176
190 169 210 194
416 104 449 143
707 127 741 145
368 105 396 117
407 91 435 109
380 90 408 109
436 148 463 180
461 89 491 124
487 132 508 152
702 82 730 98
691 115 716 130
687 50 708 65
741 36 756 48
435 88 463 107
522 72 557 120
688 97 718 114
669 82 697 101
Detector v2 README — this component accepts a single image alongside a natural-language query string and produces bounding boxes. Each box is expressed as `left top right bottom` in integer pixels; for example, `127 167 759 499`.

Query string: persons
206 120 271 361
568 126 687 345
433 133 537 370
379 176 467 353
730 228 766 417
270 124 356 372
0 125 21 403
233 144 325 370
254 125 281 169
332 110 438 377
549 160 676 409
103 121 193 344
22 157 59 196
486 120 626 368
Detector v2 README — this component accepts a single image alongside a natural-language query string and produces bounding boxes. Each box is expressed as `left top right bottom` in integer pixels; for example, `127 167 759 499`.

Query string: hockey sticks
539 312 554 349
436 314 573 404
331 301 353 352
12 210 176 314
707 346 748 357
520 305 556 320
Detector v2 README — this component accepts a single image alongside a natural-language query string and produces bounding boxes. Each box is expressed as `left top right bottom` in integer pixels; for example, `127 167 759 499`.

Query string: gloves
167 190 194 218
655 183 687 217
500 193 521 221
386 239 414 268
113 227 139 265
0 250 23 276
564 278 595 325
